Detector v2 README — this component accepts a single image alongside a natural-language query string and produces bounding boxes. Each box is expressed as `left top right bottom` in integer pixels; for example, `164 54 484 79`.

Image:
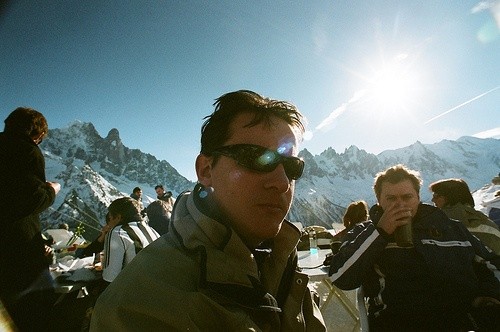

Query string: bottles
308 227 318 254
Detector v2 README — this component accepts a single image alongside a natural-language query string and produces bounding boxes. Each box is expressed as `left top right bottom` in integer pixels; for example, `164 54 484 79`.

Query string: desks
49 259 103 310
297 249 360 321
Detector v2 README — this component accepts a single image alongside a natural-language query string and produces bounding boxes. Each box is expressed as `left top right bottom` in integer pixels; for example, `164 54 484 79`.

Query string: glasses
208 143 305 180
432 192 445 199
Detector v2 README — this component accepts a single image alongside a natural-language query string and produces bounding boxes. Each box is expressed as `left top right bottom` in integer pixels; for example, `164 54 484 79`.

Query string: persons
42 185 177 310
429 178 500 256
328 164 500 332
343 201 368 229
0 106 60 332
88 90 327 332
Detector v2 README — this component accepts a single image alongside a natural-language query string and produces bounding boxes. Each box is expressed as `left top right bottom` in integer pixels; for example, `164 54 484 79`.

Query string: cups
395 210 414 247
